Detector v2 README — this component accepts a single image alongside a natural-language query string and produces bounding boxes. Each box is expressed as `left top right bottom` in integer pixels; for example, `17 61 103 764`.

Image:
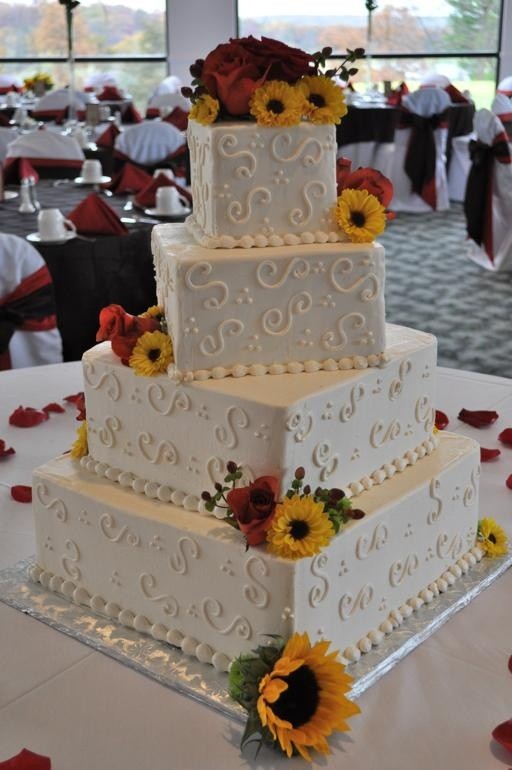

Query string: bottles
19 177 41 216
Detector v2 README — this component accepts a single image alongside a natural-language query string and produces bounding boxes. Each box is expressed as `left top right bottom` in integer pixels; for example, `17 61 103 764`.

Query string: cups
38 209 75 237
2 83 117 143
154 185 190 213
82 159 103 179
152 169 176 182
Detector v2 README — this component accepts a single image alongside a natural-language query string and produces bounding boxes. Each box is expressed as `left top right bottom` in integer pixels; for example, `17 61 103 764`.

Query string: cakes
23 35 486 685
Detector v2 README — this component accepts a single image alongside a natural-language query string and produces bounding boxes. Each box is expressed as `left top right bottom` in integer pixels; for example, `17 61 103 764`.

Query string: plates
74 175 113 186
144 206 192 219
3 190 19 201
25 231 77 246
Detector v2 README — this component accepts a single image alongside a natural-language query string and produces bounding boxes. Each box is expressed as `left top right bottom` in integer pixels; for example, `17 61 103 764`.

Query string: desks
335 85 512 278
0 74 193 369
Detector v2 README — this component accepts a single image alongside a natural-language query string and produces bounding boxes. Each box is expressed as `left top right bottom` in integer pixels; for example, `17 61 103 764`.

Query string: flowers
95 304 173 375
179 36 368 126
474 512 508 565
199 460 365 565
222 627 372 760
